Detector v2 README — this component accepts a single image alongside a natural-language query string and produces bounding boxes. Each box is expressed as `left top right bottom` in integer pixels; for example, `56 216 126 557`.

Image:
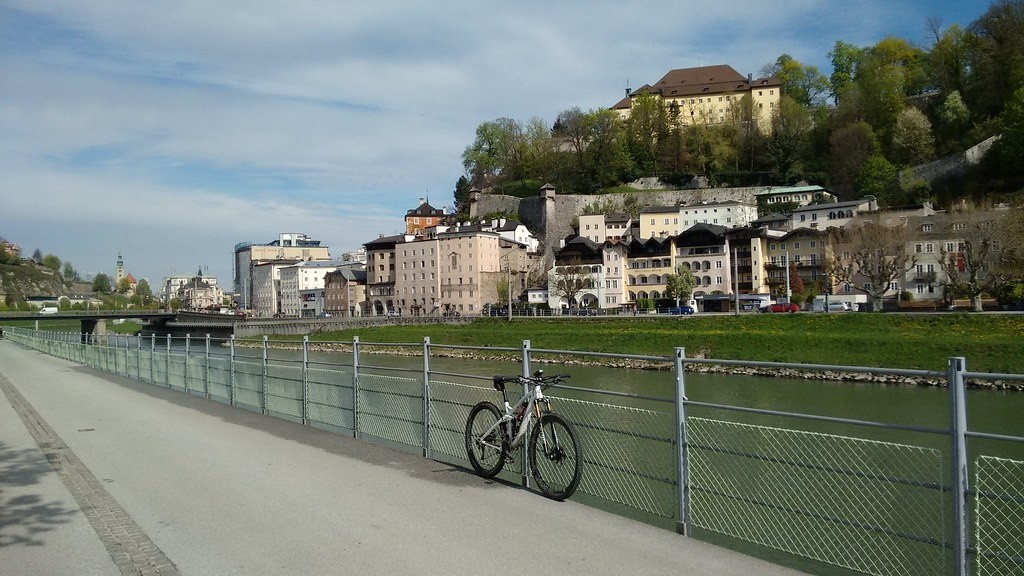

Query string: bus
220 305 229 314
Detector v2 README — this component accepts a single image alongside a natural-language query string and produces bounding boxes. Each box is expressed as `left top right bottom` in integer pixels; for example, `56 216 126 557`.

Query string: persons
634 304 637 316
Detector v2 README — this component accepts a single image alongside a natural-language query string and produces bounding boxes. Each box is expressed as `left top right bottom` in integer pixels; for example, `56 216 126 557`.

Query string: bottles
517 401 529 421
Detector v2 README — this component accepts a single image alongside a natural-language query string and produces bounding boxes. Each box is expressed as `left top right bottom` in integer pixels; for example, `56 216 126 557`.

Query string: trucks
38 308 58 315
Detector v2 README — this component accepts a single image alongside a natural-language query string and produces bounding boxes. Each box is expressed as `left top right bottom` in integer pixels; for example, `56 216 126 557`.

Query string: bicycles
465 370 583 501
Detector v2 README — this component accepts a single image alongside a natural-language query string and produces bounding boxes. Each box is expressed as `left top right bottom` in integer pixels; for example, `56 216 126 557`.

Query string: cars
576 308 597 317
273 312 285 319
771 303 799 313
441 311 460 317
319 311 332 318
387 311 400 317
757 305 770 313
225 310 235 315
670 306 694 315
823 301 851 311
